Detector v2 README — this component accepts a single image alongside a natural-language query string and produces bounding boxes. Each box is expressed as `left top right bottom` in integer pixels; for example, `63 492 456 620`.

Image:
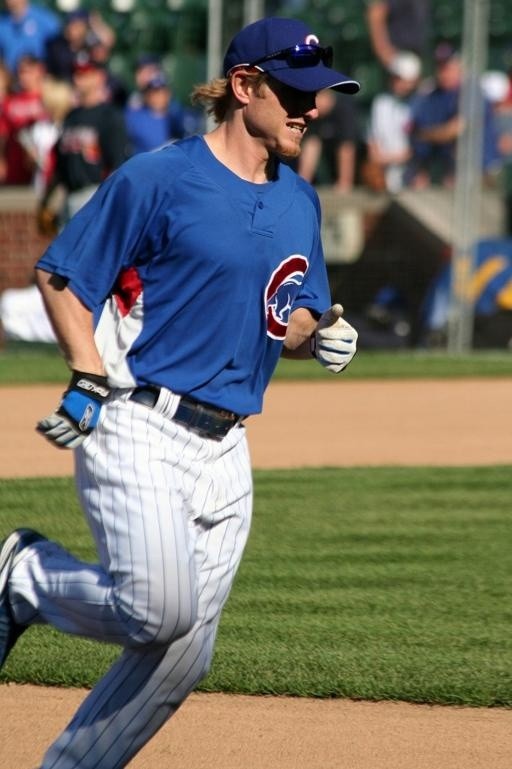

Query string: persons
1 15 371 768
4 2 510 347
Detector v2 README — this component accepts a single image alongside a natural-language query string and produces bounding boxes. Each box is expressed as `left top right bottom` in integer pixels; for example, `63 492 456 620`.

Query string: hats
224 17 362 94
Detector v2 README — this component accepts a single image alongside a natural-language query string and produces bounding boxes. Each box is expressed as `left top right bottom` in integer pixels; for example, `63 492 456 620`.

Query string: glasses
245 44 333 71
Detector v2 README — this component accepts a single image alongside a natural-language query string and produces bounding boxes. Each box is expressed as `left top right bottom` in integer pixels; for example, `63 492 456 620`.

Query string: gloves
36 370 112 450
310 304 359 374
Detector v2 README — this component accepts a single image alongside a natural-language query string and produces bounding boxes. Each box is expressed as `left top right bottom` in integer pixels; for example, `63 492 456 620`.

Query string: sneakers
0 529 48 671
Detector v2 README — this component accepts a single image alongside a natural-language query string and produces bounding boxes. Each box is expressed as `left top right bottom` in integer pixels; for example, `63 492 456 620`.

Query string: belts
129 386 234 438
